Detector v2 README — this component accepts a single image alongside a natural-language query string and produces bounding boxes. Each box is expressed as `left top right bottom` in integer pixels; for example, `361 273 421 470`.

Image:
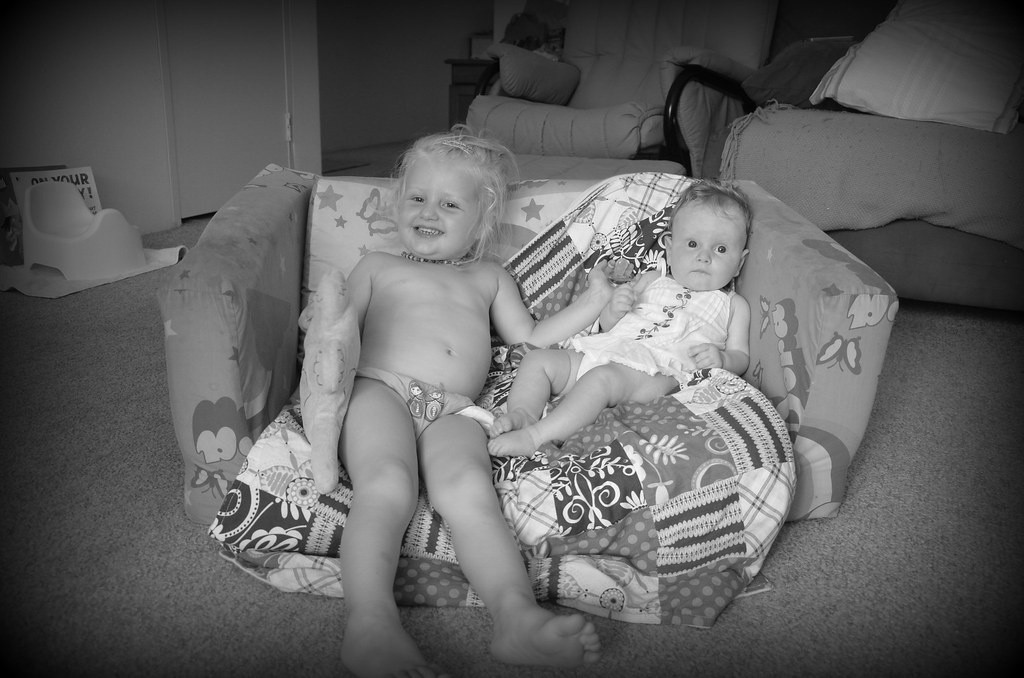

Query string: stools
22 182 145 280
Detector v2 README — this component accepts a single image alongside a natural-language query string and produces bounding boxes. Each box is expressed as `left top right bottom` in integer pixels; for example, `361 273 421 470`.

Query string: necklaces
401 250 471 264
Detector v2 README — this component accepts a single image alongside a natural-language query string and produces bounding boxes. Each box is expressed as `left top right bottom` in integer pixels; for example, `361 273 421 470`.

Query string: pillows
489 44 581 106
808 0 1024 137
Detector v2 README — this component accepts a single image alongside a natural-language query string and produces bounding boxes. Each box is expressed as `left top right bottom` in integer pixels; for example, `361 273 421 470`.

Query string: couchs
665 0 1024 313
160 162 900 627
468 0 779 178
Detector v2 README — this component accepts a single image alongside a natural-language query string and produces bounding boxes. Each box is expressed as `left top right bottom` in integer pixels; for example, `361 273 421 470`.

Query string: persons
487 180 749 457
297 132 620 678
